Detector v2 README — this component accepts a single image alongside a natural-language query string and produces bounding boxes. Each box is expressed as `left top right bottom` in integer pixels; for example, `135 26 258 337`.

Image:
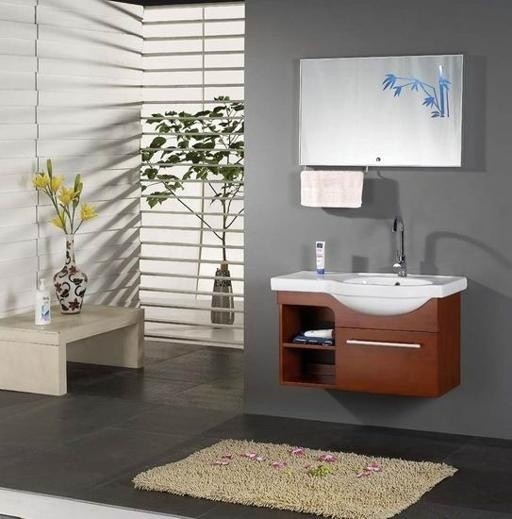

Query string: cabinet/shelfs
278 291 460 398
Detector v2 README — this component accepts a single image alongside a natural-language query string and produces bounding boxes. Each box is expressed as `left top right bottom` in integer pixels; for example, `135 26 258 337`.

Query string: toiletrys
315 241 325 275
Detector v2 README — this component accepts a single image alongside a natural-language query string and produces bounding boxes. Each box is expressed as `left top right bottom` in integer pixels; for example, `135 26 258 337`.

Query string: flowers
31 159 100 235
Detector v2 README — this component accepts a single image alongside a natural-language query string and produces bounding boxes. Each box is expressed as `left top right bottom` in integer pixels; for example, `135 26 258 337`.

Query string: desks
0 304 144 396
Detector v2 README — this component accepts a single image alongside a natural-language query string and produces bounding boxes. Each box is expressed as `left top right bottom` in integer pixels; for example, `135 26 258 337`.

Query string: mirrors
298 53 464 169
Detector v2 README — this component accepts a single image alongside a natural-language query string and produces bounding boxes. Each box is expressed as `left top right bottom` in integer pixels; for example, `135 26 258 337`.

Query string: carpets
131 439 459 518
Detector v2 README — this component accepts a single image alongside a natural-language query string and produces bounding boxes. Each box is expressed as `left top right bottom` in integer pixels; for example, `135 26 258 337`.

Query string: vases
54 234 88 314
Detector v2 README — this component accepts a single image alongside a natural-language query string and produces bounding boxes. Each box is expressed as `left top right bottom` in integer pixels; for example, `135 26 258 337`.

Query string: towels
301 170 363 208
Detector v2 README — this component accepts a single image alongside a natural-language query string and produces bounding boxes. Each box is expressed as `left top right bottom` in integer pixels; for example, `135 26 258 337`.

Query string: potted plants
141 97 244 329
